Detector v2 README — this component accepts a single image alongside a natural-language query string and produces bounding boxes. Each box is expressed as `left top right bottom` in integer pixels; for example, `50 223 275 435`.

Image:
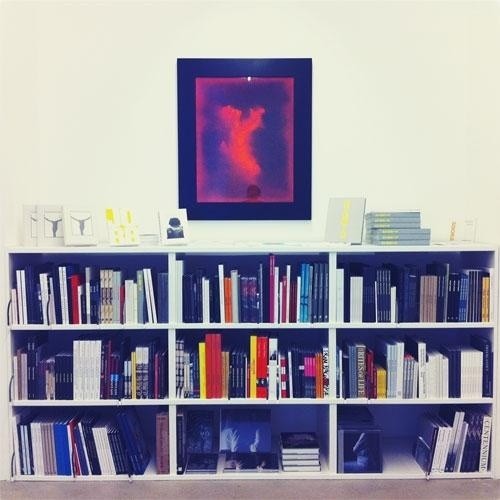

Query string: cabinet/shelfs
2 244 497 478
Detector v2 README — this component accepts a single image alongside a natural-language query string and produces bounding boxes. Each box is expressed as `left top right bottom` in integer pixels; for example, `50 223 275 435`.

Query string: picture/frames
62 207 98 246
23 203 38 247
36 205 65 247
177 58 311 220
156 208 190 246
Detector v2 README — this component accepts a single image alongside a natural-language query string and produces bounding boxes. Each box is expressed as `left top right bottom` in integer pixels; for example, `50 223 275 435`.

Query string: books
338 404 384 473
14 337 167 400
337 261 490 324
279 431 321 472
365 211 431 246
12 410 170 478
176 334 329 400
337 336 493 400
411 405 492 476
10 265 168 325
176 256 329 323
176 413 187 475
